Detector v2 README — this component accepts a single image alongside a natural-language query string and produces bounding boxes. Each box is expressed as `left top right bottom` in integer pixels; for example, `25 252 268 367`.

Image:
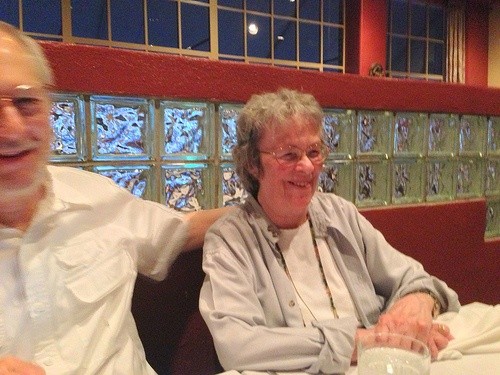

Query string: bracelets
404 288 441 319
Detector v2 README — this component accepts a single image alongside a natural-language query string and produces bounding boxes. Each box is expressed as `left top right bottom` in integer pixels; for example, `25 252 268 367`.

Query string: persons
0 20 245 375
199 89 461 375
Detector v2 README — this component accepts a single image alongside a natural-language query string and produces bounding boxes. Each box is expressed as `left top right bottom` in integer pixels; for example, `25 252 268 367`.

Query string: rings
438 322 445 336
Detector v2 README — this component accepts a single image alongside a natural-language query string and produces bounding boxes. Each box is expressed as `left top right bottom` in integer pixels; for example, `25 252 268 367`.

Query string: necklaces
272 213 340 322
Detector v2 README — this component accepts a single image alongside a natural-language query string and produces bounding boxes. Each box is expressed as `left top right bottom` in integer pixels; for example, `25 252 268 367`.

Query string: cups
356 333 431 375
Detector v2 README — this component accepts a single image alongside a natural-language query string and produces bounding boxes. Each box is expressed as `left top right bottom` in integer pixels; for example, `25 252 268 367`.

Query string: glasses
0 85 54 115
259 144 330 166
